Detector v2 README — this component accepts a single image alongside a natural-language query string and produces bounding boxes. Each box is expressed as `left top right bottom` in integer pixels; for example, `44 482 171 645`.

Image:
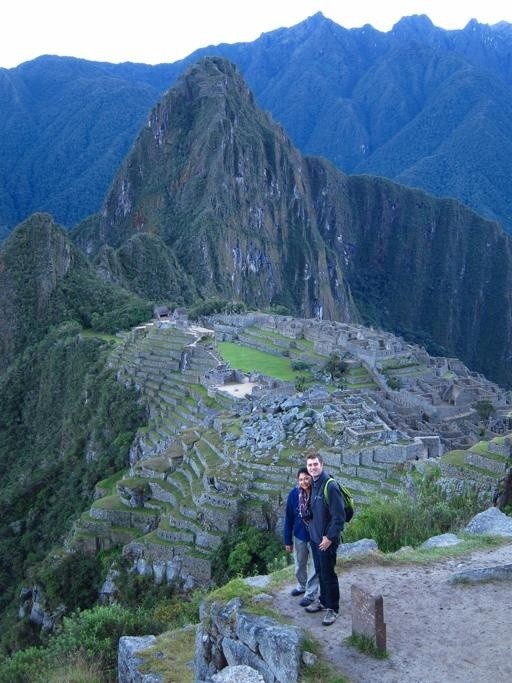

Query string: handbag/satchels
324 476 354 521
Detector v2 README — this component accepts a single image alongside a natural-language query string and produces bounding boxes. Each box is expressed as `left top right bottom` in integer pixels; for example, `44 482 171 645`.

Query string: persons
284 467 320 606
304 452 347 626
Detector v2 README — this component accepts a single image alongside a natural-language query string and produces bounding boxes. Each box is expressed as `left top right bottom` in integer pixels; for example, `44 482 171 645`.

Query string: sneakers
322 608 339 625
291 588 306 595
305 600 326 612
299 595 315 606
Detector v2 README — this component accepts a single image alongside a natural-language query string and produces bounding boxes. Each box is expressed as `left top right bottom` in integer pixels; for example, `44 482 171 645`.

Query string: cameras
302 510 313 521
